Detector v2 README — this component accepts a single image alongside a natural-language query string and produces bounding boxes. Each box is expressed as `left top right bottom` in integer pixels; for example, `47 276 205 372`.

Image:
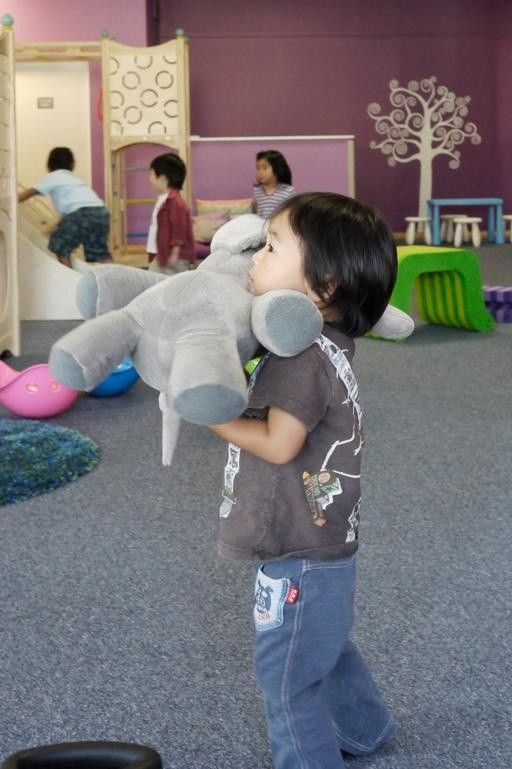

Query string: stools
400 208 512 250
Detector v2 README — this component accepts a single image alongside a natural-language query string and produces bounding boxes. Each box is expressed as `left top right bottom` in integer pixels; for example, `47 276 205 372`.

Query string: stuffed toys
48 213 415 468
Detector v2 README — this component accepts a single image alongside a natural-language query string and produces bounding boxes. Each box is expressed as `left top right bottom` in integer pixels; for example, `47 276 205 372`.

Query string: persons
146 153 195 273
206 190 399 769
251 150 296 219
18 147 112 268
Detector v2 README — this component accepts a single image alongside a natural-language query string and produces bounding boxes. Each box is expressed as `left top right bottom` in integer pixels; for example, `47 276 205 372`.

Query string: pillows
188 205 232 247
191 195 258 223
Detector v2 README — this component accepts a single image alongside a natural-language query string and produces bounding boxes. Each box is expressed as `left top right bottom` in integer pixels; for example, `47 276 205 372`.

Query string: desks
426 196 506 249
